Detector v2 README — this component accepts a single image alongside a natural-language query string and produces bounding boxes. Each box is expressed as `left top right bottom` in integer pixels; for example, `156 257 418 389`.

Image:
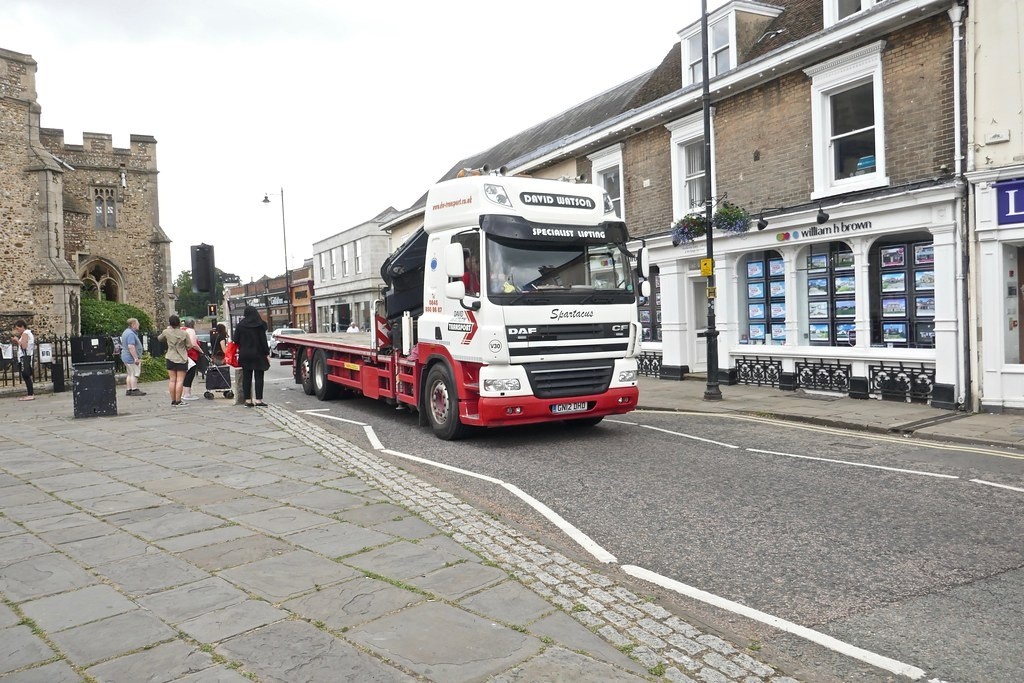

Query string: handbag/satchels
224 342 241 367
213 355 226 365
20 346 33 378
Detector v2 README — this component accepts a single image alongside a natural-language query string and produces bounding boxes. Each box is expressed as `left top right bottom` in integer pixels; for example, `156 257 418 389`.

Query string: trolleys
202 351 234 399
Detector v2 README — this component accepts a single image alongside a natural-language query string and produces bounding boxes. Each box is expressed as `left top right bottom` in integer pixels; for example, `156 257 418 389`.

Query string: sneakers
245 402 254 407
19 393 36 401
126 389 146 396
256 402 268 408
171 400 188 407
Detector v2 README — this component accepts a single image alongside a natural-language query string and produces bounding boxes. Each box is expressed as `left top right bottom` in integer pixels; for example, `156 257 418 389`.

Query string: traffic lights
208 304 217 318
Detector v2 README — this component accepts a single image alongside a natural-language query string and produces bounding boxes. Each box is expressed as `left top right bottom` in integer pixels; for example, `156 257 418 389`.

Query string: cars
269 328 307 359
266 332 272 347
197 335 211 354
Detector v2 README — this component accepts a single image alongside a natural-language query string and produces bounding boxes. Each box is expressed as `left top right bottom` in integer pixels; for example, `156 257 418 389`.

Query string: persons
157 315 193 407
233 306 271 407
11 320 35 401
462 256 479 294
180 319 228 401
346 322 359 333
121 318 147 396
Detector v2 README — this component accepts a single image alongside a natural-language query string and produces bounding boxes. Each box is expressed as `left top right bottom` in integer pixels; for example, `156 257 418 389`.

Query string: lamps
757 206 784 230
817 195 847 224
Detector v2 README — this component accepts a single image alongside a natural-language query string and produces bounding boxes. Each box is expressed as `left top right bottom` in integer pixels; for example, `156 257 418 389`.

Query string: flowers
670 215 705 247
713 201 751 240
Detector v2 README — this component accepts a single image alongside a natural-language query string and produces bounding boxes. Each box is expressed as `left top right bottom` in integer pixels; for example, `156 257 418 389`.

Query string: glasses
472 260 480 264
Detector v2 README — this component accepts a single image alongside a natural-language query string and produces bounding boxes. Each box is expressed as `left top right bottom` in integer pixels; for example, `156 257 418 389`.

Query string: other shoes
181 395 199 400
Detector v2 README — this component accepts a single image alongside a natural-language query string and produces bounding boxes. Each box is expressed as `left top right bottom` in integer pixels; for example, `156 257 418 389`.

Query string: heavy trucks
273 163 651 440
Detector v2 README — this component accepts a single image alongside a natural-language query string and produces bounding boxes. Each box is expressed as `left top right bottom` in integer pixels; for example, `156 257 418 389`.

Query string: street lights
261 187 292 328
